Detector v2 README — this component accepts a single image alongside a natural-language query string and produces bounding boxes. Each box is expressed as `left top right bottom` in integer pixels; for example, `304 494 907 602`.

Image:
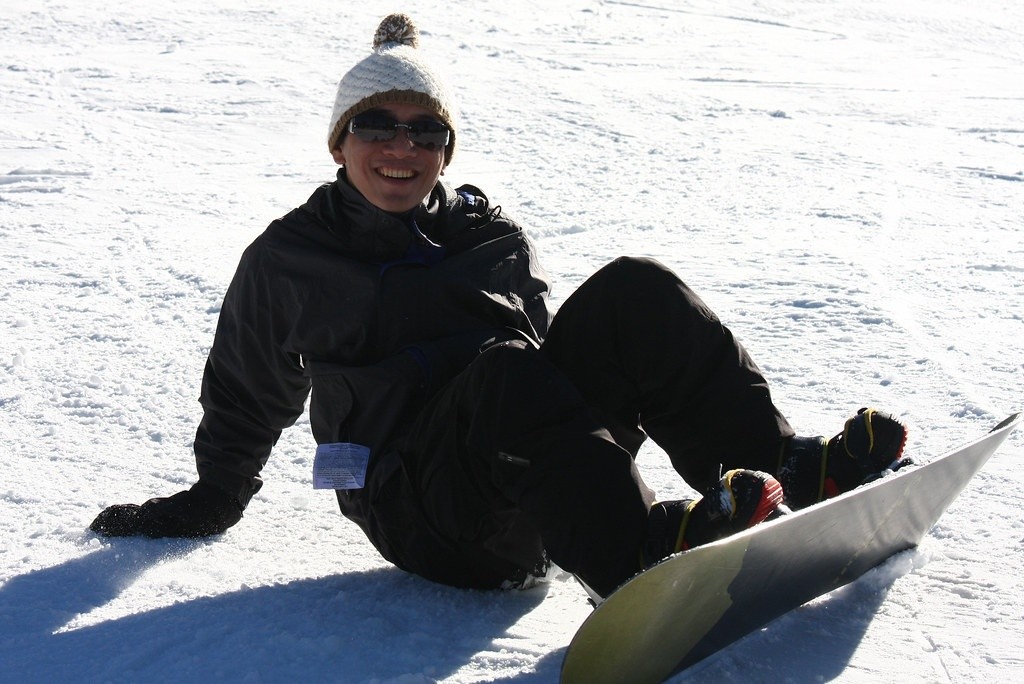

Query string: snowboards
557 408 1022 684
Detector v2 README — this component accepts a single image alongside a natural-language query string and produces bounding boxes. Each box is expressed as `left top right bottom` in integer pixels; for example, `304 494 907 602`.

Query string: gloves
88 471 264 543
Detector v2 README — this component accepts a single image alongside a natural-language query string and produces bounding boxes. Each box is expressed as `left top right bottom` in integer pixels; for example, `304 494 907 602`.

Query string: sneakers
772 411 905 512
648 464 785 557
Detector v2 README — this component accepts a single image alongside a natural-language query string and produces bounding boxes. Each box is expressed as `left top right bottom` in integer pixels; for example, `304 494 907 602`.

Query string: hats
329 16 454 166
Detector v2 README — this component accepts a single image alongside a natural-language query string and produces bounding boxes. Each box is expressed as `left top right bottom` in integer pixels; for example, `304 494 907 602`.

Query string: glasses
349 109 450 151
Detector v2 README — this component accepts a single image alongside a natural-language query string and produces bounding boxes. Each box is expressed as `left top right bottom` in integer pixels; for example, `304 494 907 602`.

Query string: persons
89 14 916 605
